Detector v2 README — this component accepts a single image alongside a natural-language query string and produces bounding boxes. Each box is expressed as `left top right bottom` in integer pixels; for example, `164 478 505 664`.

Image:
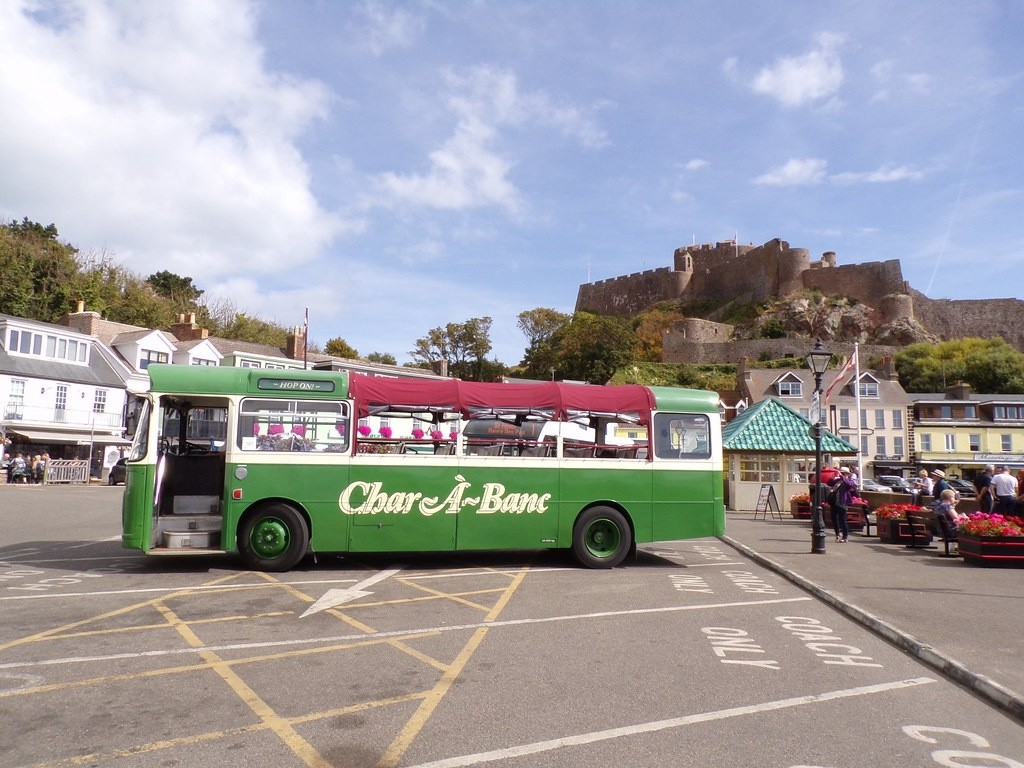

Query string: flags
824 350 856 405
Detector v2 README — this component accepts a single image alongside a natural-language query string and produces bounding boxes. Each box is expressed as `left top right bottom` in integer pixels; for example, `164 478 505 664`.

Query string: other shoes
840 538 848 543
835 536 840 542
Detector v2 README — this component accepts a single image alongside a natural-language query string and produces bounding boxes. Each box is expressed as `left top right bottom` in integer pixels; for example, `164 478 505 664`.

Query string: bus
461 414 634 459
122 362 726 573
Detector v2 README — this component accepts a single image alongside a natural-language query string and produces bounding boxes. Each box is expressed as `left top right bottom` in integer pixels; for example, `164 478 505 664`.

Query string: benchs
9 461 73 485
339 441 639 459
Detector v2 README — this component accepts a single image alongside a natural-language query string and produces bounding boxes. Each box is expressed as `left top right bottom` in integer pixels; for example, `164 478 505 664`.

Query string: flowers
820 496 870 508
292 426 306 436
449 431 458 440
874 503 931 522
956 508 1024 536
411 429 425 439
335 424 346 433
379 426 392 438
359 424 371 436
254 423 258 436
267 424 285 435
430 431 444 439
790 491 810 506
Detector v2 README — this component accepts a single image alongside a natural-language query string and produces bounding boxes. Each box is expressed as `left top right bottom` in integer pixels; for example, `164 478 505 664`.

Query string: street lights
805 336 833 554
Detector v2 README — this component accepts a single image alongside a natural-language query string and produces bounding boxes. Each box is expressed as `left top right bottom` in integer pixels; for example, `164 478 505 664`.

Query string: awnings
874 462 916 469
10 428 133 446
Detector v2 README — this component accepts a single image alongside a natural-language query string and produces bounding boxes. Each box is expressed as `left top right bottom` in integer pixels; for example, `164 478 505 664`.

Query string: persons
0 450 47 484
973 464 995 514
990 465 1024 515
912 470 934 496
849 466 859 489
827 467 854 542
931 469 948 499
934 489 960 528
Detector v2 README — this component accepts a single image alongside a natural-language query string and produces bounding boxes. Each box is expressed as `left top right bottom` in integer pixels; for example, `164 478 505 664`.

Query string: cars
861 474 977 498
109 457 128 486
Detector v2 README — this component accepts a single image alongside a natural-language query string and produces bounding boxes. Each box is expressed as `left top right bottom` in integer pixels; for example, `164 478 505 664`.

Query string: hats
930 469 945 479
837 467 851 474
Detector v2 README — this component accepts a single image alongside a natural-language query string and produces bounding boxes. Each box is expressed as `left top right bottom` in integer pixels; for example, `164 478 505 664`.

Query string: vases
791 504 814 520
957 533 1024 569
822 507 866 532
876 516 933 545
